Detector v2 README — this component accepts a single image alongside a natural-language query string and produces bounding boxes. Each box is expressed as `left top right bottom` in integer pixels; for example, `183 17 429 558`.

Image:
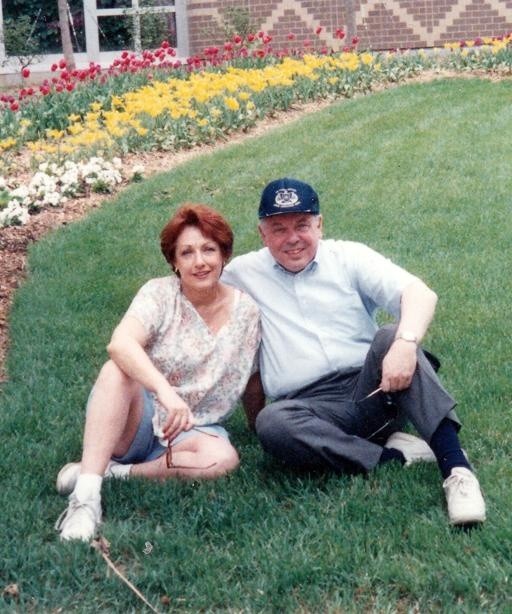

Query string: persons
217 176 488 527
54 201 261 543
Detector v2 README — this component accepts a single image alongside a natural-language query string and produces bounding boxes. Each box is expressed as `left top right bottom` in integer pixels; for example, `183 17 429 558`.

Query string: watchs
393 330 420 346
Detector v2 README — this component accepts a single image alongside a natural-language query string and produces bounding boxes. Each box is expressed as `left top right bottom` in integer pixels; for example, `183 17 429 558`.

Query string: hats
258 179 319 218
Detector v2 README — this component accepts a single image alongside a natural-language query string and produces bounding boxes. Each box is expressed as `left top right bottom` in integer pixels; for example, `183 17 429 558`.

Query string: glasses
356 388 395 439
167 428 218 469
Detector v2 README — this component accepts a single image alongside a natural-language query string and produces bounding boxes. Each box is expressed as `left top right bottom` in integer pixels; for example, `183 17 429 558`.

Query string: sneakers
55 492 102 543
385 433 468 467
444 467 486 524
56 457 117 495
